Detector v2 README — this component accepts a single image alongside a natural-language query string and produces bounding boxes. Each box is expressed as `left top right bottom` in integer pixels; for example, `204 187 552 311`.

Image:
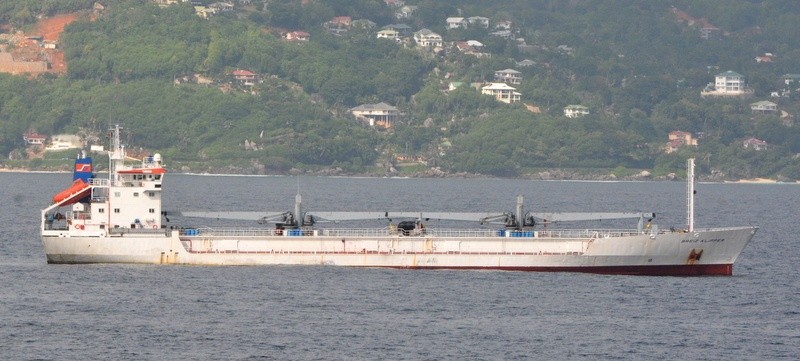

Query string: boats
39 125 758 281
